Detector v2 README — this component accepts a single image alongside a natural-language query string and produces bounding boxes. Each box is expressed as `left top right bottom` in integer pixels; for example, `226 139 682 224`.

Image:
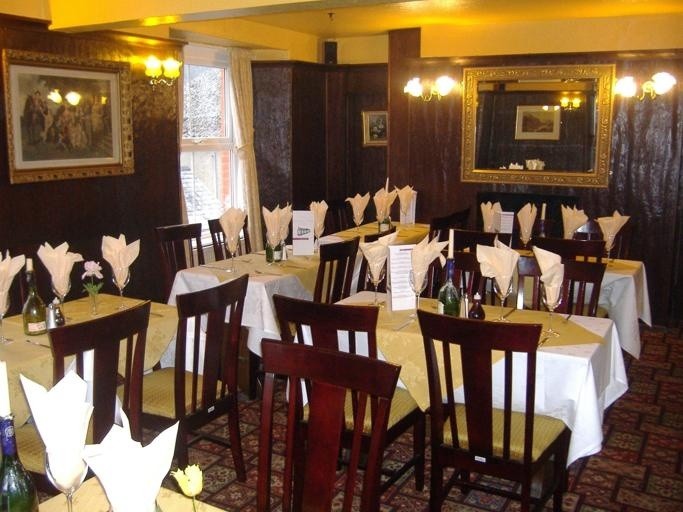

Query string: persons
9 65 120 164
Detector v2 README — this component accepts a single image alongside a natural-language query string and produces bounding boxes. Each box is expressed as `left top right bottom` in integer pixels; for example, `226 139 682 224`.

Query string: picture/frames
515 103 562 143
1 47 137 185
359 107 390 149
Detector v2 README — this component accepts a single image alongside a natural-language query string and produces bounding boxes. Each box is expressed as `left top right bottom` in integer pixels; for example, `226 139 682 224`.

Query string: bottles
538 219 546 238
460 293 469 319
468 291 486 320
437 257 460 317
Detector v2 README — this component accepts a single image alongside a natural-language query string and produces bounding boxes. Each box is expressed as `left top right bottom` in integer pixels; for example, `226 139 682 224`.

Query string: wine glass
279 226 289 249
353 214 364 233
378 210 388 218
542 285 563 338
111 267 131 311
315 226 324 249
520 229 532 249
603 236 615 267
225 236 239 270
0 289 13 345
492 277 513 322
267 233 280 266
409 270 428 308
50 274 72 323
45 452 88 511
367 264 386 308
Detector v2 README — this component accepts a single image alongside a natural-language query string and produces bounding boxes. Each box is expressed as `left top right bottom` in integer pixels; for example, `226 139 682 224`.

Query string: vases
89 292 99 314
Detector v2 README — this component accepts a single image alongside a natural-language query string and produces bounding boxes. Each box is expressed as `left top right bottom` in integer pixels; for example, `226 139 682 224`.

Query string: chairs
272 293 427 505
479 210 548 253
443 227 512 265
530 234 605 267
424 248 511 313
256 338 403 512
10 298 150 501
426 205 472 247
308 234 362 307
516 253 609 318
116 272 249 491
151 221 203 302
309 196 376 235
357 226 399 295
562 212 634 261
416 309 572 512
207 214 252 263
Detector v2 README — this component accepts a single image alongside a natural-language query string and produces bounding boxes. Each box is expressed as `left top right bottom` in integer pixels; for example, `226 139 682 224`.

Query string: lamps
542 104 562 112
140 54 183 94
612 71 677 103
401 75 459 103
45 88 83 108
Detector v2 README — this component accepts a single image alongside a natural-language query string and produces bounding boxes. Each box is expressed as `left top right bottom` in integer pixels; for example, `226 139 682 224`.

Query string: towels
372 177 397 223
345 192 370 224
262 203 293 246
101 234 140 286
359 231 398 282
561 204 589 239
593 210 631 251
397 185 415 216
18 370 95 488
476 234 520 297
410 230 450 292
532 246 564 306
0 249 25 315
219 206 247 252
480 201 502 233
37 241 84 295
309 199 328 234
517 202 537 244
83 407 179 512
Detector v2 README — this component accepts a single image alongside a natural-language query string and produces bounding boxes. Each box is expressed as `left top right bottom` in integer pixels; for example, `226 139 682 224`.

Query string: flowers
82 262 105 292
169 464 203 512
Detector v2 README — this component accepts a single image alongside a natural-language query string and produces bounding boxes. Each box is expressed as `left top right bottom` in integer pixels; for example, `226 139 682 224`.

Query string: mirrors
459 64 615 189
